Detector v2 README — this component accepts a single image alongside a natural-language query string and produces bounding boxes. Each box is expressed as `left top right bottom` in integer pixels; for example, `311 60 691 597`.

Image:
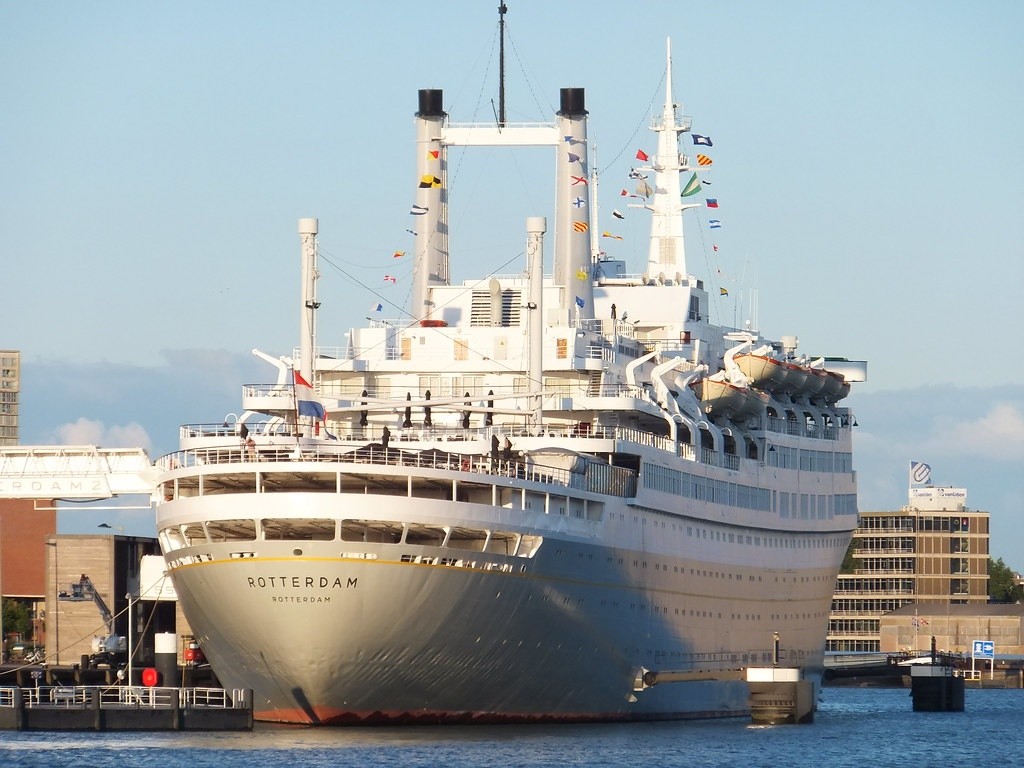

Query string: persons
245 437 256 463
241 424 249 449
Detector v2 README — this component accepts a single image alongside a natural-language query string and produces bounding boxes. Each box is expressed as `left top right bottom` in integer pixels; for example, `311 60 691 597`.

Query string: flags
425 150 439 160
681 172 702 197
418 174 443 189
912 617 929 632
371 302 382 313
708 219 721 229
384 275 396 285
409 205 428 216
628 167 648 180
294 369 338 441
636 149 648 162
706 198 718 208
720 287 728 297
691 134 713 146
564 135 590 309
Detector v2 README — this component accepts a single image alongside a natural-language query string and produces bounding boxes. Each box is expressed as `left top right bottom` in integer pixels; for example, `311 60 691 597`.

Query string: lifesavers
463 461 469 471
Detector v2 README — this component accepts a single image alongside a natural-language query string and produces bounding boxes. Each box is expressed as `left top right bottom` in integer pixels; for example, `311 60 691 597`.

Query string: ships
152 1 867 723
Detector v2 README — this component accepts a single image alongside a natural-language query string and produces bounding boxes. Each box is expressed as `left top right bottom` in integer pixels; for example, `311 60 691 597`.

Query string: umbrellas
491 435 512 464
401 390 435 428
461 390 494 429
344 427 465 464
359 389 369 427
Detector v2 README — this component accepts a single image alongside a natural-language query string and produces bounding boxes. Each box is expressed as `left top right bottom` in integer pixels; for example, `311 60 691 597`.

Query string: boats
643 632 818 725
777 358 852 401
733 352 779 388
737 387 770 423
822 638 967 714
690 382 739 416
